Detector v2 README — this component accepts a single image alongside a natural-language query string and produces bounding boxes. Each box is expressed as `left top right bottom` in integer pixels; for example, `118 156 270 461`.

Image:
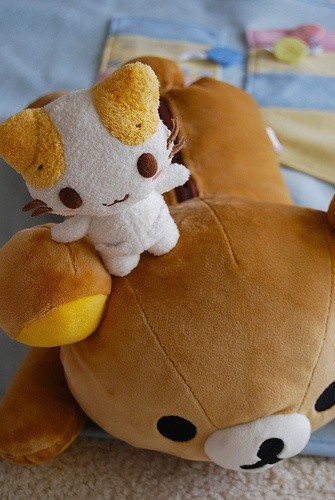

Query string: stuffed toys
0 62 191 277
0 55 335 474
